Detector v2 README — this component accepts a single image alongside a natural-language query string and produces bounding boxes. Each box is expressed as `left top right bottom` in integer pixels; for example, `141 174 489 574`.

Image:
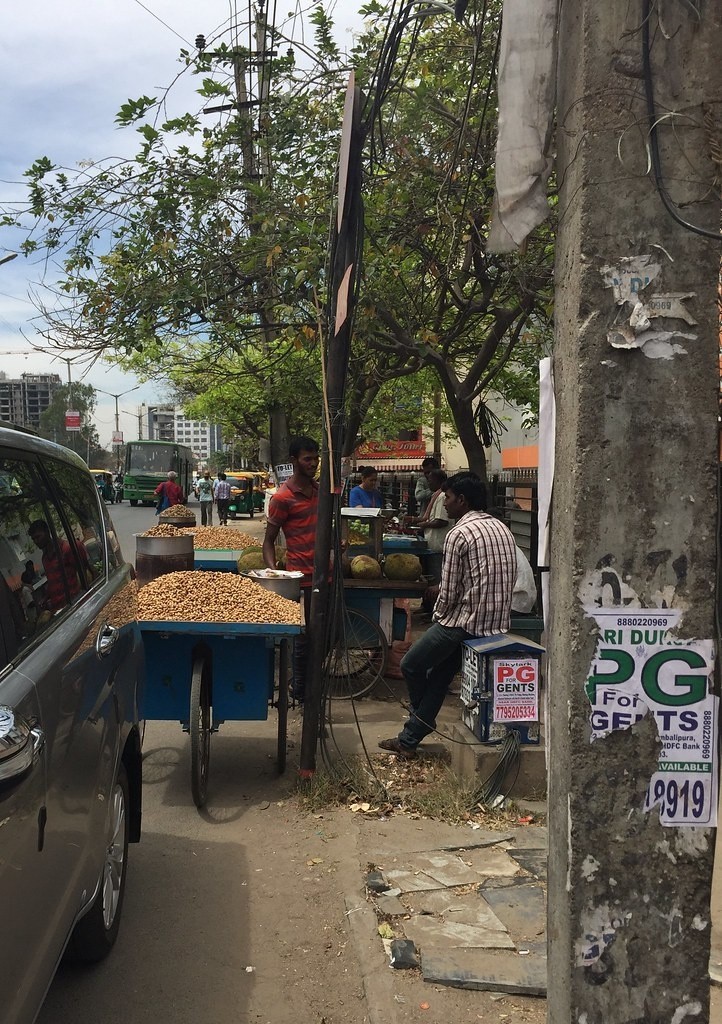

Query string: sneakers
378 737 416 760
297 701 305 718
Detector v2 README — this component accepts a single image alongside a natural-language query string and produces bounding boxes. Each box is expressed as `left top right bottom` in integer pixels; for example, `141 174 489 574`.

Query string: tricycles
140 558 290 810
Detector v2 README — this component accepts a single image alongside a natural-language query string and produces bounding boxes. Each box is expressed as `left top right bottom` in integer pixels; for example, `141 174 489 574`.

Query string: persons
114 472 123 485
511 546 537 617
215 474 231 525
94 475 104 486
349 466 383 508
154 471 184 514
213 473 222 490
193 473 201 498
415 457 440 516
263 436 334 701
196 469 215 525
5 514 110 627
412 469 456 621
379 471 517 757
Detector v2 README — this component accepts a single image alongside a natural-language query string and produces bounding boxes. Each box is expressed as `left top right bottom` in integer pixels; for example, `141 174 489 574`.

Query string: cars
1 425 145 1023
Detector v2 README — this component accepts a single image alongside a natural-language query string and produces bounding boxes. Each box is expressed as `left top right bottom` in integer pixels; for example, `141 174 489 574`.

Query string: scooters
192 481 200 501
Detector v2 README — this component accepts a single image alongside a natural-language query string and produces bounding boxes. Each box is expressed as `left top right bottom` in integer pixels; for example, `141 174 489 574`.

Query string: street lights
93 385 140 471
121 409 157 441
143 424 172 441
29 345 112 449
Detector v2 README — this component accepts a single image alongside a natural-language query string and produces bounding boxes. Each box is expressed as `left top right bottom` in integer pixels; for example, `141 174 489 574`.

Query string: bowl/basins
240 569 304 602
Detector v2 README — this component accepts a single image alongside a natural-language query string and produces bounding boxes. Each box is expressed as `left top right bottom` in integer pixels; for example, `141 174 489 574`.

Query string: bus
124 439 194 506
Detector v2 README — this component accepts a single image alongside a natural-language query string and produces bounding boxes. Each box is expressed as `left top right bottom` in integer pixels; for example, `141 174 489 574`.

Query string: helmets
98 474 102 478
95 474 100 479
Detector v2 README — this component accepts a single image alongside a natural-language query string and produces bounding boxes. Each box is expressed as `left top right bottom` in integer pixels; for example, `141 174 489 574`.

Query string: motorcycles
111 481 124 504
89 470 116 504
247 472 263 512
222 472 255 519
258 471 275 508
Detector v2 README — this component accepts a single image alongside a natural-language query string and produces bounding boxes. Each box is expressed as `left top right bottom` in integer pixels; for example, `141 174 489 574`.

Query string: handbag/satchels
155 495 170 517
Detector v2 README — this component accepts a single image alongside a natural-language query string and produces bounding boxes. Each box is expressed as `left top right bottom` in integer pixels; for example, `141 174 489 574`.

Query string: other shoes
224 523 228 526
219 521 223 525
